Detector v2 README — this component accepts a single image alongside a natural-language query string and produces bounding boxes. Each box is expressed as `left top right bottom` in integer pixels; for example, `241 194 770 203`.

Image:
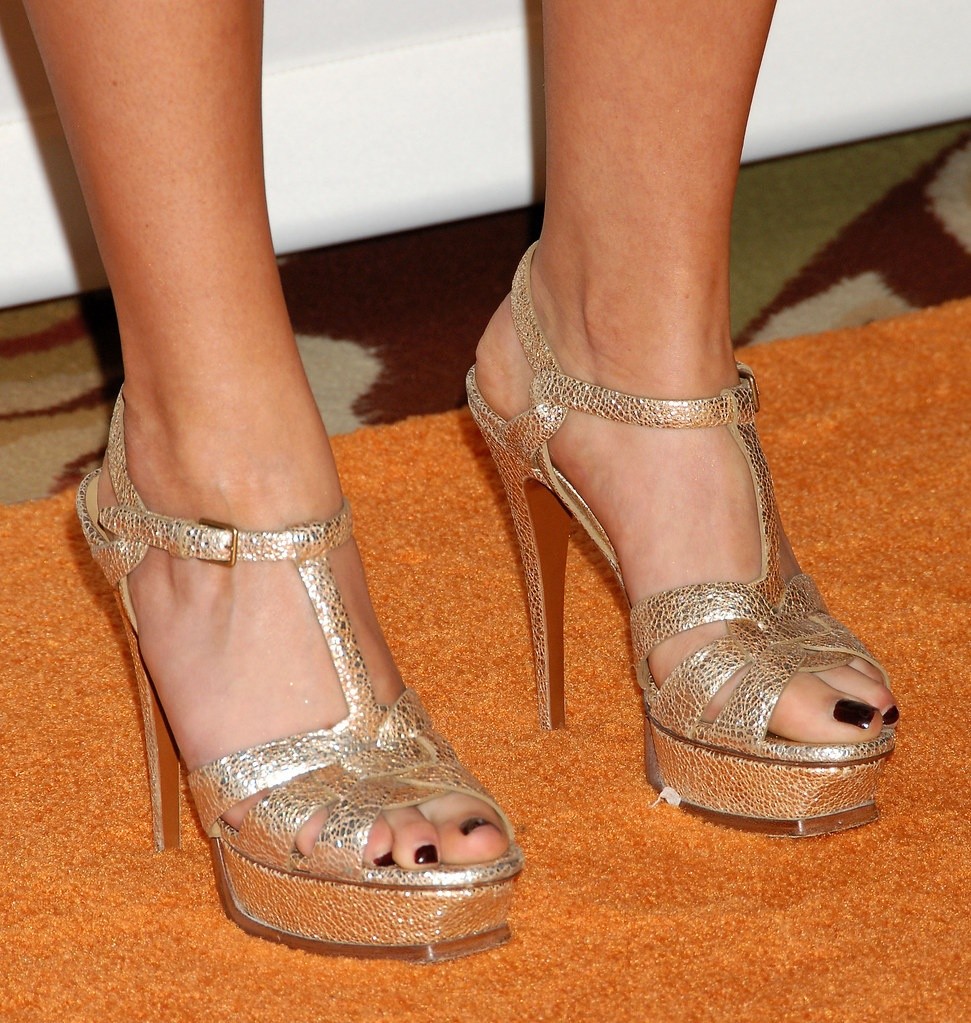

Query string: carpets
0 284 971 1023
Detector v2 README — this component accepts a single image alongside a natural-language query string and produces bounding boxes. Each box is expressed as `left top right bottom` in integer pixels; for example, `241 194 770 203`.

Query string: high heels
465 239 896 838
75 379 522 963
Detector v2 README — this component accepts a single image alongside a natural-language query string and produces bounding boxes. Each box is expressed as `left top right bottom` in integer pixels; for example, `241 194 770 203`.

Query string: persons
28 1 901 963
